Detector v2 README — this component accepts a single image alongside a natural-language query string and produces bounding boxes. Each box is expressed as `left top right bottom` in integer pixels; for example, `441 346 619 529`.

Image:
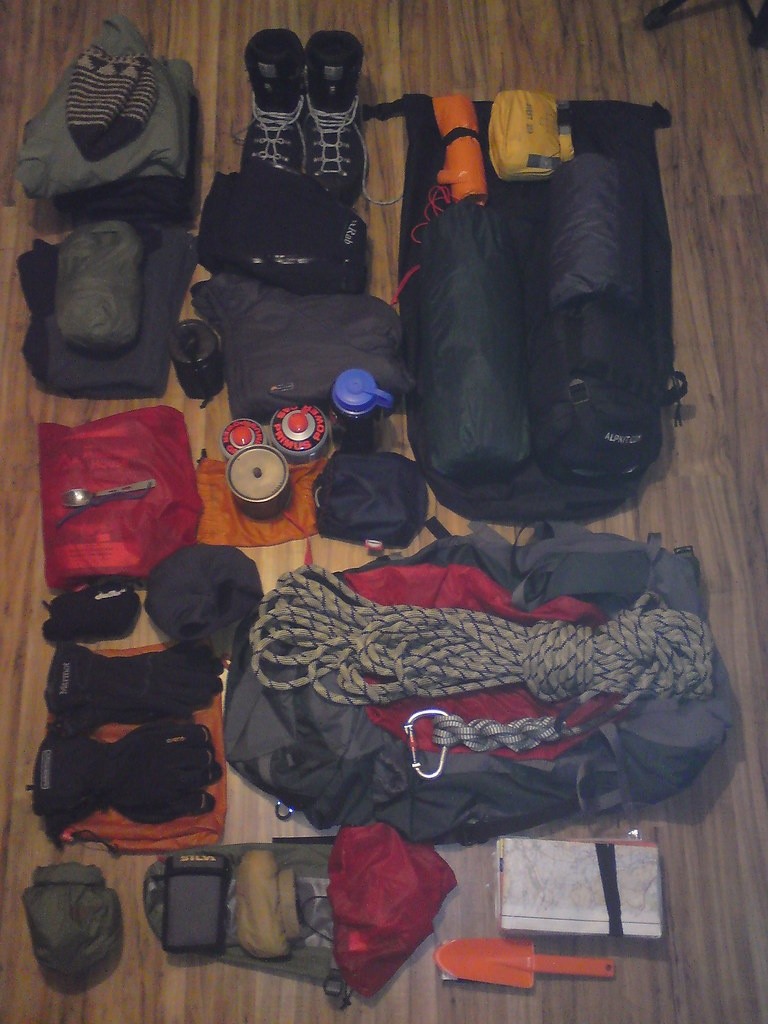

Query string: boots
240 28 304 175
305 30 369 207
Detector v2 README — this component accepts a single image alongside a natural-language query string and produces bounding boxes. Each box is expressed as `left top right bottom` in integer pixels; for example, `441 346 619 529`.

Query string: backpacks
227 520 739 846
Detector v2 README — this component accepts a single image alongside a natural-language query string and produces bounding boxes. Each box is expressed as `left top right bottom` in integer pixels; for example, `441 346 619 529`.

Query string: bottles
169 319 223 399
327 368 393 453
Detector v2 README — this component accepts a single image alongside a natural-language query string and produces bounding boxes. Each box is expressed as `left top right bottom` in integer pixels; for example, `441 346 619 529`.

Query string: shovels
431 936 617 990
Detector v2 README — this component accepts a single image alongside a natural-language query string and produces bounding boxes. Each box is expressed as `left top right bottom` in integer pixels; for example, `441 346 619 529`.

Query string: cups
225 444 294 519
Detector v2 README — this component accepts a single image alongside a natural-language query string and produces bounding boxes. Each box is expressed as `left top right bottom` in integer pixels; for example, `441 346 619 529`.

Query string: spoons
61 478 156 506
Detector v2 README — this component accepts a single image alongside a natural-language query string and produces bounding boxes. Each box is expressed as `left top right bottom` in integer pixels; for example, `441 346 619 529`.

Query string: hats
64 44 156 163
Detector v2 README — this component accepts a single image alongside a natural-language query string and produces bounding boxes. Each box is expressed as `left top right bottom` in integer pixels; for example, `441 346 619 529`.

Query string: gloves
44 639 225 738
26 716 222 853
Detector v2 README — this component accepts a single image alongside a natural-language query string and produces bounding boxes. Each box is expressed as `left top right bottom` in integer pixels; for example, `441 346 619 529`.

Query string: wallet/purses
162 852 232 957
